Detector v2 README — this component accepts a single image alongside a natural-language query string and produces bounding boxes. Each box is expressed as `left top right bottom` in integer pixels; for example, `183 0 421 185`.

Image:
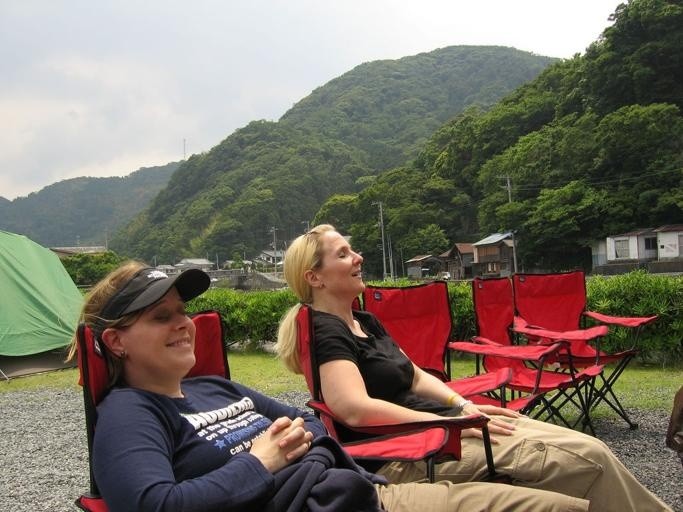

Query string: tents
0 230 89 380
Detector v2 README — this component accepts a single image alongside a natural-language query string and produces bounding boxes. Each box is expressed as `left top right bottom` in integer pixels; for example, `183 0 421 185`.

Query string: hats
94 267 210 355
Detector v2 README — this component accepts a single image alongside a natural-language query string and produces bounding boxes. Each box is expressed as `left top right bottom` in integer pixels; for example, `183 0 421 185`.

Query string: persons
62 260 589 512
276 223 674 512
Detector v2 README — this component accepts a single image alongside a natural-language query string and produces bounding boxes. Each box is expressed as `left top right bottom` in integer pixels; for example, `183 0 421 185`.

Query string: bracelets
455 399 473 408
447 390 459 406
